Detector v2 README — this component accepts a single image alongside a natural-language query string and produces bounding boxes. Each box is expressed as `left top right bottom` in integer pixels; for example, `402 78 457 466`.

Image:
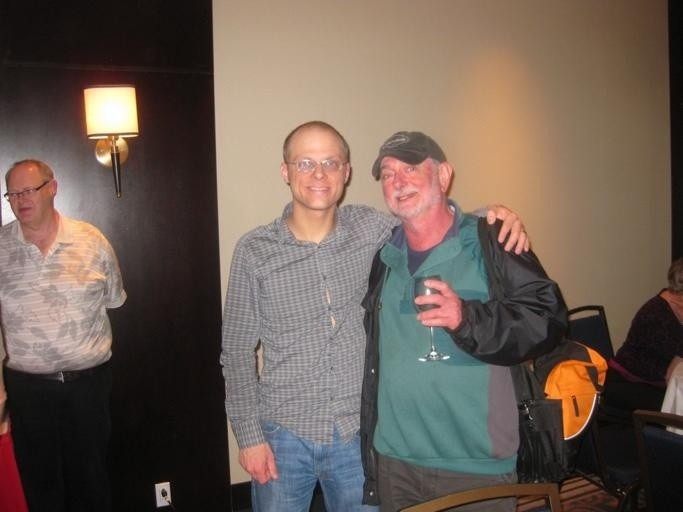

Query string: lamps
82 83 140 197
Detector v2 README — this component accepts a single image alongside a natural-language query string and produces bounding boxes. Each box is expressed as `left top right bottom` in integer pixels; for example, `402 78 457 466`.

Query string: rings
519 229 527 234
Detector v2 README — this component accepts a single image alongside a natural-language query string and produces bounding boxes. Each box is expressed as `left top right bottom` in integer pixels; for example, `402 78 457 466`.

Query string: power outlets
154 481 172 508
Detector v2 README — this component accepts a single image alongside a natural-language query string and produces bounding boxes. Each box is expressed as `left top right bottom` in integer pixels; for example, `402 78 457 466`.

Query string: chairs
634 409 683 512
565 305 615 362
398 482 561 512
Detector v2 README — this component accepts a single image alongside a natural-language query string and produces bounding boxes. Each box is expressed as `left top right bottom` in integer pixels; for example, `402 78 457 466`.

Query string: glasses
4 180 53 201
283 158 349 174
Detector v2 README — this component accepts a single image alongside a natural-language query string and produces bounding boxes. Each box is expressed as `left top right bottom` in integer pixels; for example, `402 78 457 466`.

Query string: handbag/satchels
477 217 627 490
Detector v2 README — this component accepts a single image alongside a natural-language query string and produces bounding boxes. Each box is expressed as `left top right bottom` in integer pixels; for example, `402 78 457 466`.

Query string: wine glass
412 274 452 364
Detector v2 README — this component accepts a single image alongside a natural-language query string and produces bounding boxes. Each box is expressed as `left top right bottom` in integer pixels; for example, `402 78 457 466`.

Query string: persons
218 120 532 512
598 256 683 426
658 362 683 437
1 159 128 512
360 128 569 511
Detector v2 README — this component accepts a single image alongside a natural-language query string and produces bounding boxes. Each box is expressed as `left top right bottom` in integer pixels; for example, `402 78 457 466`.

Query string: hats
371 130 448 181
